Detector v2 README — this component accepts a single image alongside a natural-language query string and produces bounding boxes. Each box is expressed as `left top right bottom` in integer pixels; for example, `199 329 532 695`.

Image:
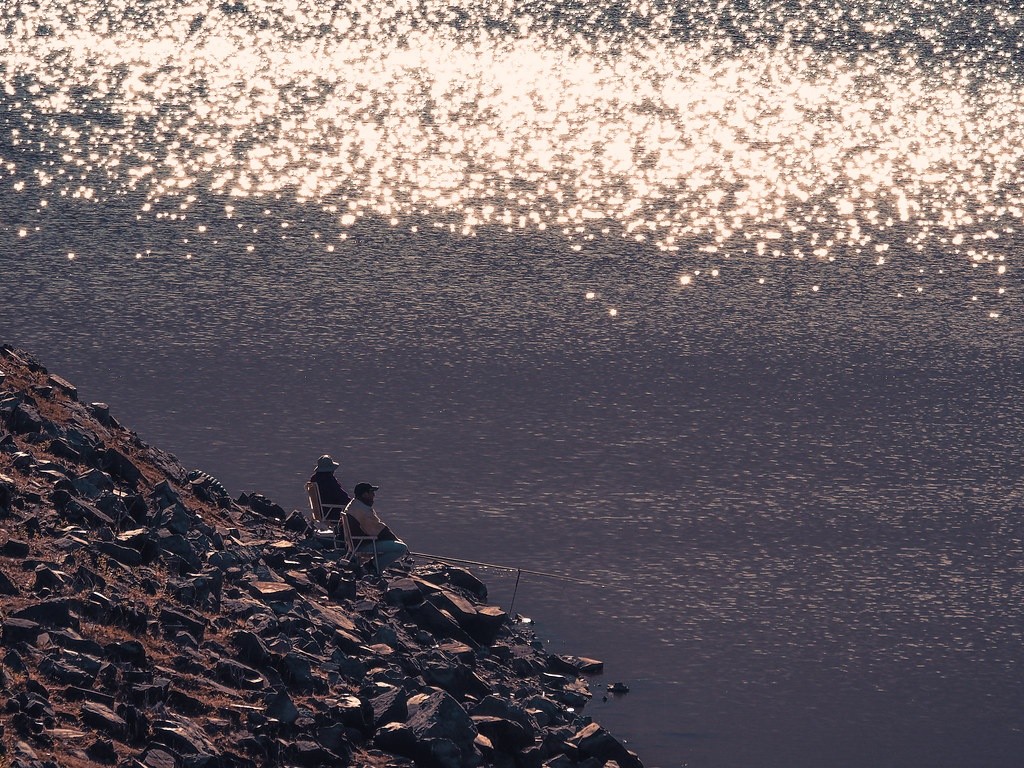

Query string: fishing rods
411 550 586 581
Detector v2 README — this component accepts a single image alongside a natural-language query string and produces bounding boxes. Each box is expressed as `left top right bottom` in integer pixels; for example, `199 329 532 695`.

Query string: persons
311 455 352 547
338 482 408 577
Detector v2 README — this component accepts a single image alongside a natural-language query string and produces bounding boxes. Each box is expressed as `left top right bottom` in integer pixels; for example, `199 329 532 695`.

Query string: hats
313 455 340 472
354 482 379 494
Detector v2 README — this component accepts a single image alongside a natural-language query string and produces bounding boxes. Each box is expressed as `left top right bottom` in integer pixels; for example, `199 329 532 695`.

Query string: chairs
302 481 347 550
340 512 385 571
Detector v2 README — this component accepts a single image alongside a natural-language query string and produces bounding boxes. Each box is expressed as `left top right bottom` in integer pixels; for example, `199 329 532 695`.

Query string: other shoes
364 559 380 575
